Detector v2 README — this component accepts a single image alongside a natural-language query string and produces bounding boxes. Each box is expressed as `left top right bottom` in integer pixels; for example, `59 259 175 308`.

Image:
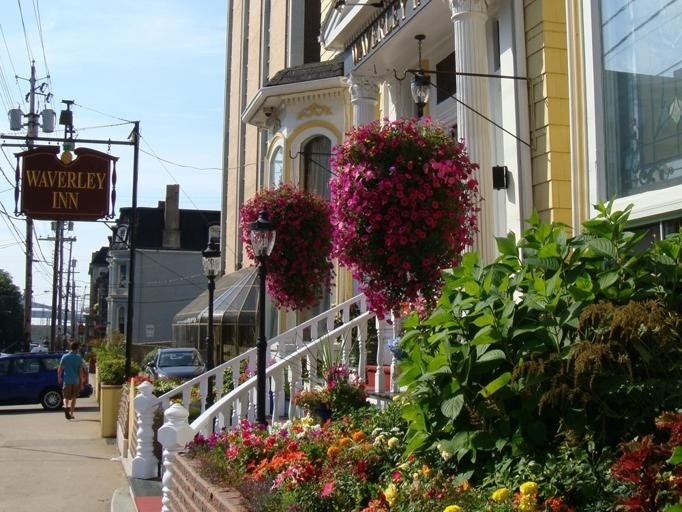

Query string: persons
56 341 86 420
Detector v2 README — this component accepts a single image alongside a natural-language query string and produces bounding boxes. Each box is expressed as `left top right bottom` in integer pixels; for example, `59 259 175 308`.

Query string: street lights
247 210 276 422
201 237 221 408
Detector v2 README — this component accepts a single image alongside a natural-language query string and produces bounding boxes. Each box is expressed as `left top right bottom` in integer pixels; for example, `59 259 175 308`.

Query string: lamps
410 33 430 119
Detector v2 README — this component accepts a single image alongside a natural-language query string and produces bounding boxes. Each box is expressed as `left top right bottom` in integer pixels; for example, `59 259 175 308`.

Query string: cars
146 347 207 380
0 352 93 412
